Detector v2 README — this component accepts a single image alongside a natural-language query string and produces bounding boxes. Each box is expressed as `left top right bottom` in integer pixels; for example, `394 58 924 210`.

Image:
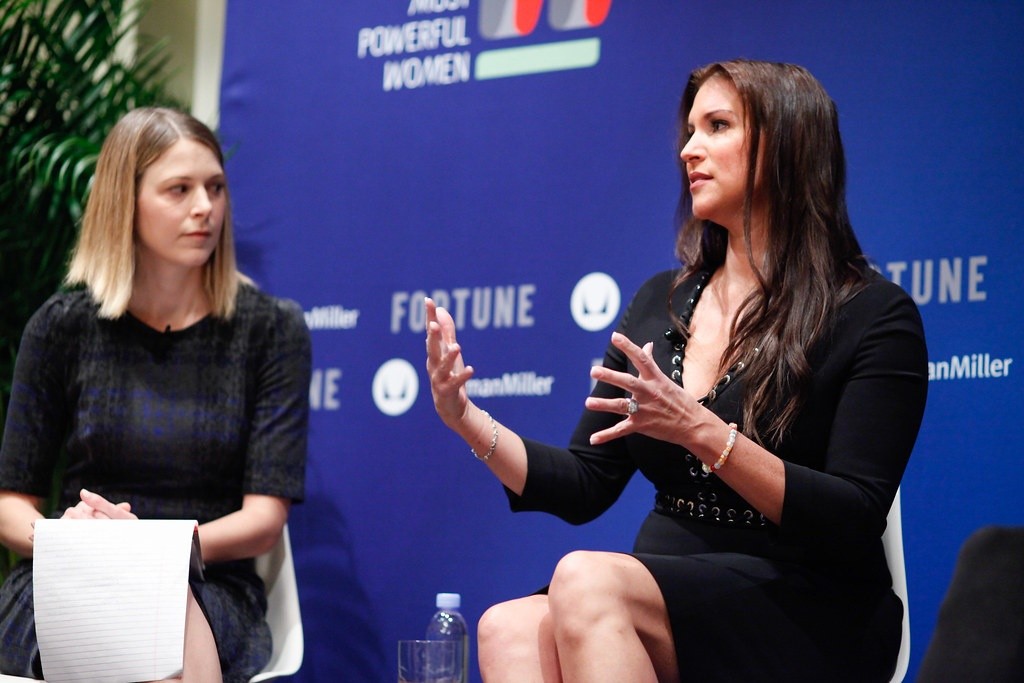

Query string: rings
625 398 639 414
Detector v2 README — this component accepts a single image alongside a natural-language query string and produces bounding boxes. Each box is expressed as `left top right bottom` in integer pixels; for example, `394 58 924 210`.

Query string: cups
397 640 457 683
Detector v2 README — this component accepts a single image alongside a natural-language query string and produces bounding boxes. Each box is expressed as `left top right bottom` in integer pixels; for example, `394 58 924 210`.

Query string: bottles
428 592 468 683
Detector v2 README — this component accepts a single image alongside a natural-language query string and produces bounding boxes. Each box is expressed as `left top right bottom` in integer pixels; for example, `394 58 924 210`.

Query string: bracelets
702 423 737 473
471 410 498 461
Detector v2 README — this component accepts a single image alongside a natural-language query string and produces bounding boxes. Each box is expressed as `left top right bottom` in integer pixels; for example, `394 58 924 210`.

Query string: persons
426 58 929 683
0 105 312 683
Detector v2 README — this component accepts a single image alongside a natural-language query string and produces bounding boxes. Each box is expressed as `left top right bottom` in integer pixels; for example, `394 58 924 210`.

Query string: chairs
247 523 304 683
884 485 911 683
